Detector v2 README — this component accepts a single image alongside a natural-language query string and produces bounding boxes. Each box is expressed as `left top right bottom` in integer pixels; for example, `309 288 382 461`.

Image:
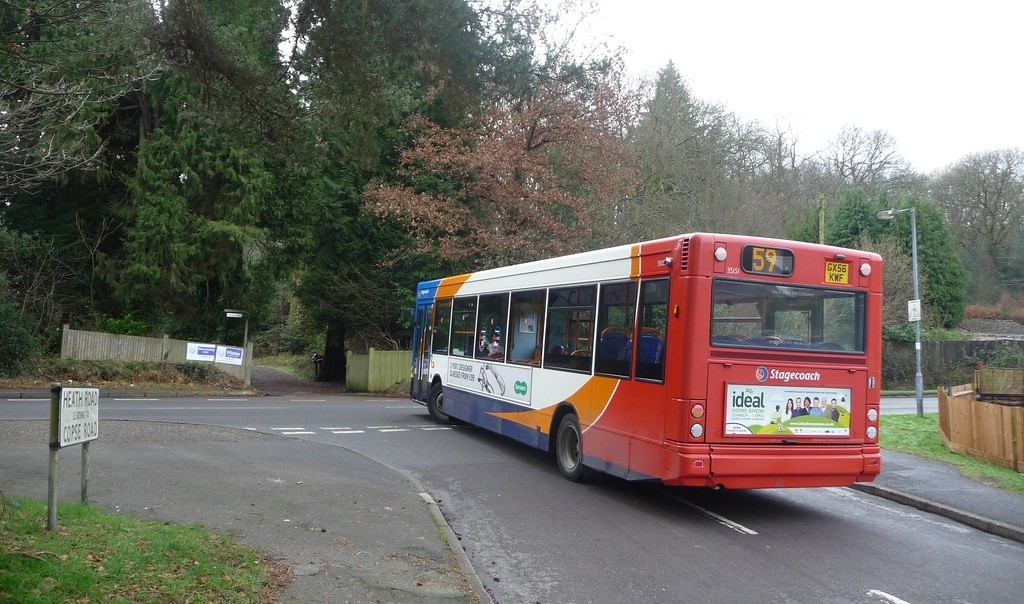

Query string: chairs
549 324 846 381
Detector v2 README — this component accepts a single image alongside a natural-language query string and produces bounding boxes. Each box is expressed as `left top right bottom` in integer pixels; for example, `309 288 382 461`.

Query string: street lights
877 206 927 418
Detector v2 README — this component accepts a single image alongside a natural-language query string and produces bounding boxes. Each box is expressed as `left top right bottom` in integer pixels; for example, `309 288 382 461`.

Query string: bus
410 233 884 491
